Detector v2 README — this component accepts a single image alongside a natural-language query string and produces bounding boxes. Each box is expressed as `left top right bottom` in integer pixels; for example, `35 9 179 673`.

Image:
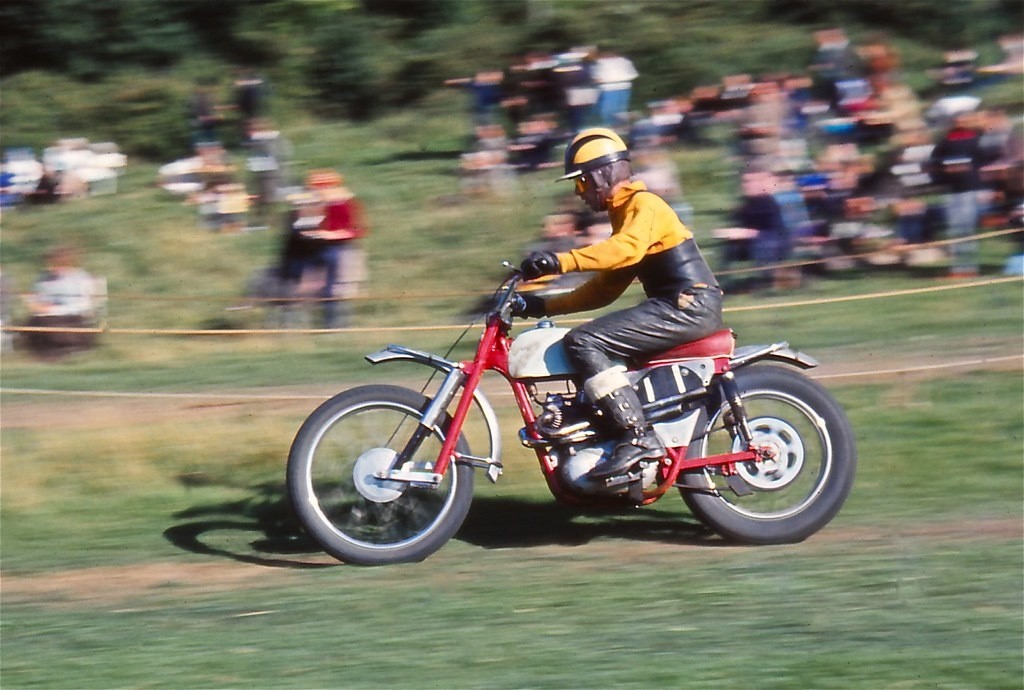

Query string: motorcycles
286 259 857 567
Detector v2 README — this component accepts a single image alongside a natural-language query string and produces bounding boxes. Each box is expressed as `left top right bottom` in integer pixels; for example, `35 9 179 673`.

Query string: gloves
520 249 561 282
510 294 548 319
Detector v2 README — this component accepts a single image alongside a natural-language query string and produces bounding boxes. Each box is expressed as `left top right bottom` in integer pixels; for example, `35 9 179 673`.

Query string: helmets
554 126 632 181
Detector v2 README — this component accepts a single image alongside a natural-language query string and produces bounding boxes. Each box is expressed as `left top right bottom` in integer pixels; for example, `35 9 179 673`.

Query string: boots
584 362 666 479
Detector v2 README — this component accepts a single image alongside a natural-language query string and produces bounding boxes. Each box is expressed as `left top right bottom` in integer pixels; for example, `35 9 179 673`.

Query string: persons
513 126 723 483
0 137 122 203
257 168 363 333
446 45 639 167
159 59 284 214
532 25 1024 293
21 250 94 340
